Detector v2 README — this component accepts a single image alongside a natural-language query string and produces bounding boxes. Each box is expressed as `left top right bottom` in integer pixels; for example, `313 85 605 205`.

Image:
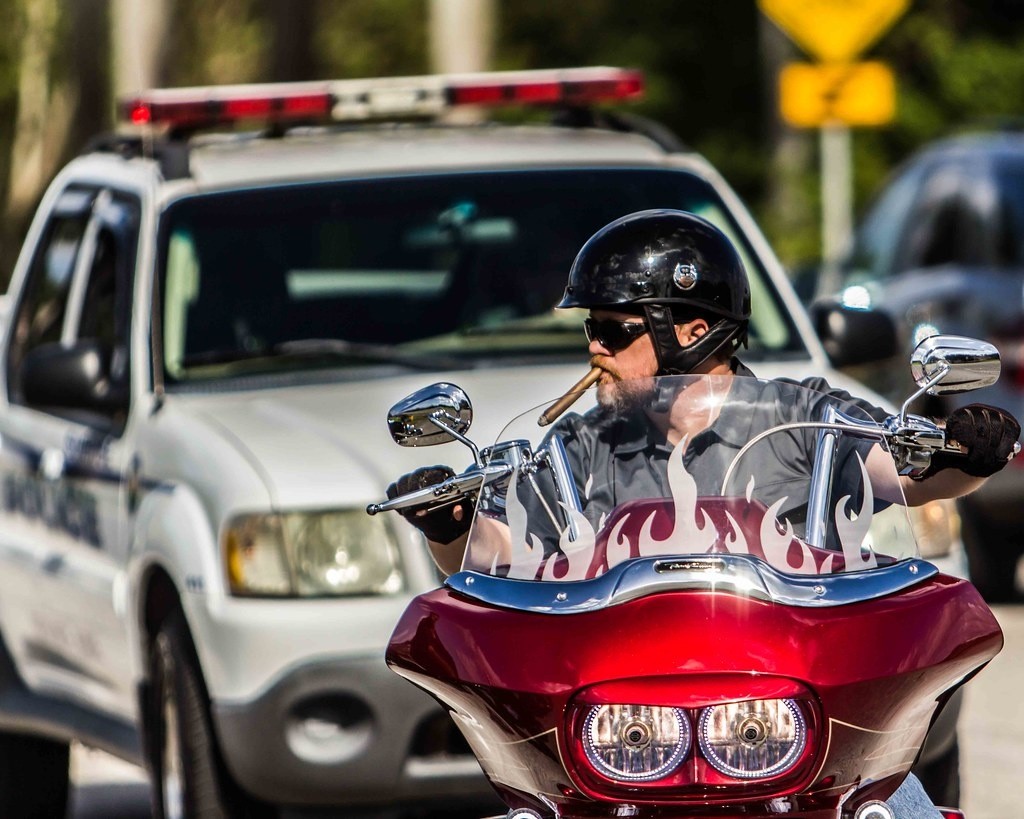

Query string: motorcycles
367 332 1022 819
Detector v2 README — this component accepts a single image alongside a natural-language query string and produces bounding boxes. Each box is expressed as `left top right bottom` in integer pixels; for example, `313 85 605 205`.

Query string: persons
386 205 1020 819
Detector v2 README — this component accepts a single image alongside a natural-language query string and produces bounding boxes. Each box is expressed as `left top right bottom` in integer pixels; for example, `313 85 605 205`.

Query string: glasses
583 317 690 351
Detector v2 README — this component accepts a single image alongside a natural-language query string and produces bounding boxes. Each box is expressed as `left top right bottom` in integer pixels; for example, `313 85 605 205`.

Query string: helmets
556 209 751 320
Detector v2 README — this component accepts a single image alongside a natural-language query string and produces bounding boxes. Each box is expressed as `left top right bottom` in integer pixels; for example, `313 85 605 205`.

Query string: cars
807 131 1024 610
2 64 896 819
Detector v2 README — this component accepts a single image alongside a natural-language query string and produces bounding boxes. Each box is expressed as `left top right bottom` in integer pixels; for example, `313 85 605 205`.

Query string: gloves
944 402 1021 477
386 465 474 546
